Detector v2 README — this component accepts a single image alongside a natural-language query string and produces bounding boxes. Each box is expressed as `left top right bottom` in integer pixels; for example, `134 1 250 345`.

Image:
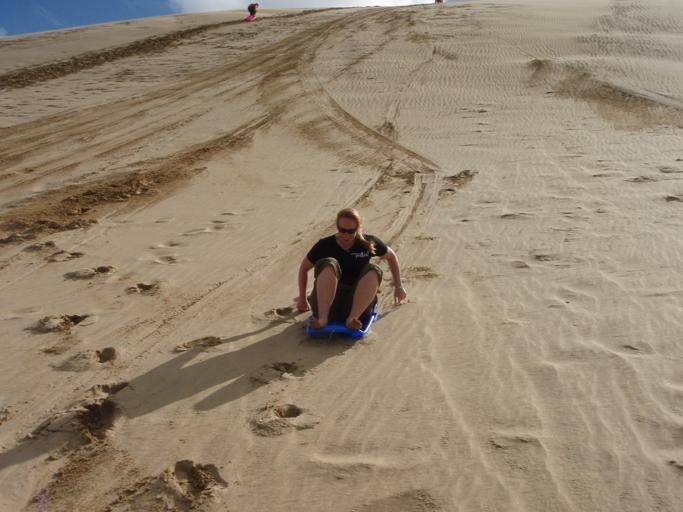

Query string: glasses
337 226 358 234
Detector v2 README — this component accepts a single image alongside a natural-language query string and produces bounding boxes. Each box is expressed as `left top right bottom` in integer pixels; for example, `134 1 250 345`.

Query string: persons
248 2 258 18
297 207 407 330
